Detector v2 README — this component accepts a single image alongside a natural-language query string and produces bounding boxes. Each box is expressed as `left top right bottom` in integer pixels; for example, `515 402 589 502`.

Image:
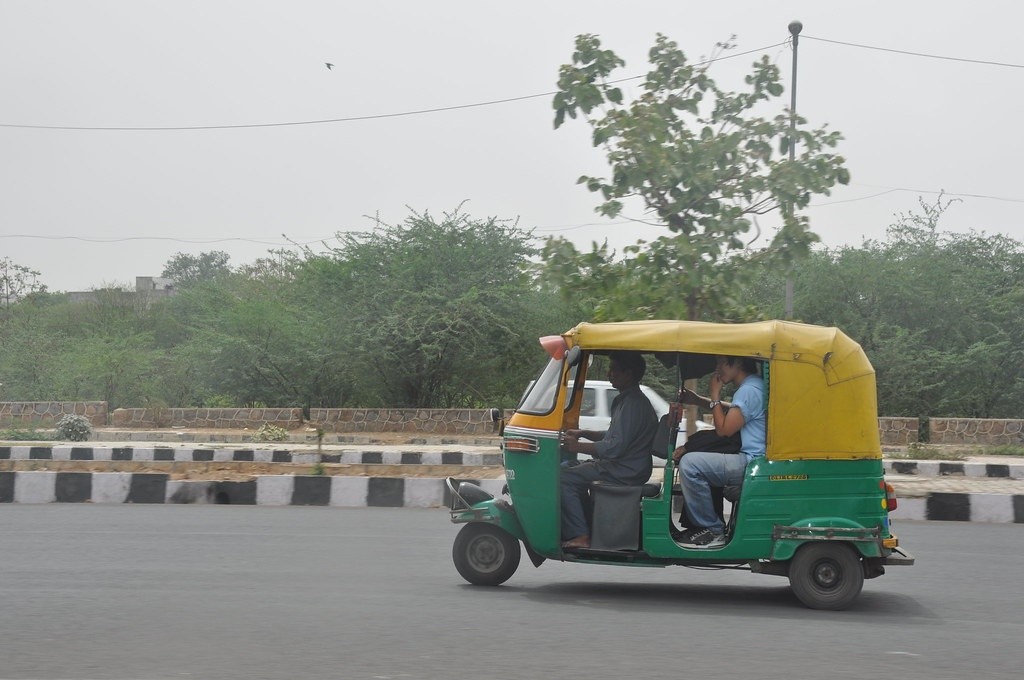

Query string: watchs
710 399 721 409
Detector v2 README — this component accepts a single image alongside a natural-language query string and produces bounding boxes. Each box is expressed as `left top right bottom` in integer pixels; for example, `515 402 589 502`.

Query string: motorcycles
447 320 915 611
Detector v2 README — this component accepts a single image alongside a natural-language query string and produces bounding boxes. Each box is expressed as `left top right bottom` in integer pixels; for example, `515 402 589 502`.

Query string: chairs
591 414 680 552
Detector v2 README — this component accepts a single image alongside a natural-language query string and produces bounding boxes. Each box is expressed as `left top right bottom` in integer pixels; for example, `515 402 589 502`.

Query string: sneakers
674 527 726 548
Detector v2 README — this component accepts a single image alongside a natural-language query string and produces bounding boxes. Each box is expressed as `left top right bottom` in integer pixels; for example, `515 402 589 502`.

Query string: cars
519 378 723 470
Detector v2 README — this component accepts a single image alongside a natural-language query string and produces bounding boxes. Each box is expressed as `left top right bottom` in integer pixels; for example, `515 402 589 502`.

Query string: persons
558 350 659 548
672 360 760 542
672 354 768 548
